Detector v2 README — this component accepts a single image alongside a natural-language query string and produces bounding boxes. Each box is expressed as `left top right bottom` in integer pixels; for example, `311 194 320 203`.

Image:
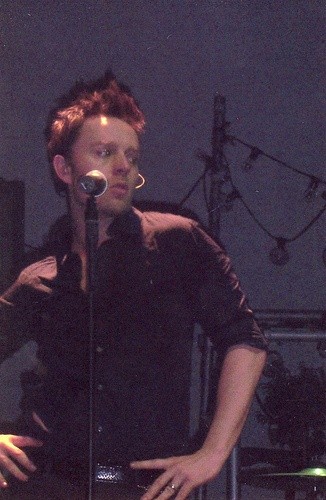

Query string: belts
33 455 167 487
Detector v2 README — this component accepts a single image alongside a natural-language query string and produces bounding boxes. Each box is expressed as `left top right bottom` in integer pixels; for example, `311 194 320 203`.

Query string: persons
0 80 267 500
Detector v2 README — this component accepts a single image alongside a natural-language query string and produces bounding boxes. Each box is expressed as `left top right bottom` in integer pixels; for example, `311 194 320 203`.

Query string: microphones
82 170 108 197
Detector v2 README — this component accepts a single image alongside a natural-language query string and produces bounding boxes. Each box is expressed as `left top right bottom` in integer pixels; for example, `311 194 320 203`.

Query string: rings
168 483 177 492
